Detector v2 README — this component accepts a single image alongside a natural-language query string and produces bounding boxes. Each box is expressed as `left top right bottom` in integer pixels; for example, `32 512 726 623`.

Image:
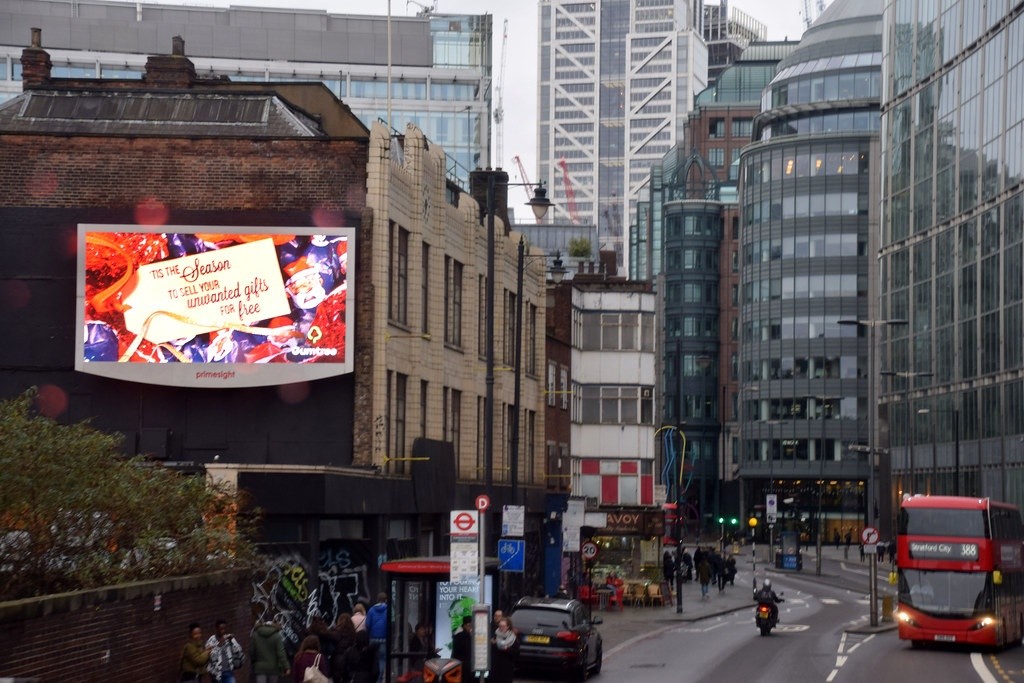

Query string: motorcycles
752 586 786 636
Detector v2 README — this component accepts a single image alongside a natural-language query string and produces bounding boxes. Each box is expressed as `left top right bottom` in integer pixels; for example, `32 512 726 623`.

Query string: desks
595 588 613 612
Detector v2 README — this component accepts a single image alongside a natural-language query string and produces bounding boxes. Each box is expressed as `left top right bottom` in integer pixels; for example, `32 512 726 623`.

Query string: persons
858 541 866 563
553 585 570 599
887 540 897 563
909 572 934 602
606 570 625 612
912 517 935 534
716 553 737 594
453 607 521 683
725 529 745 549
663 550 677 592
834 531 840 550
877 541 886 562
753 579 785 623
565 576 579 599
844 531 852 550
178 592 432 683
694 545 720 599
681 546 693 582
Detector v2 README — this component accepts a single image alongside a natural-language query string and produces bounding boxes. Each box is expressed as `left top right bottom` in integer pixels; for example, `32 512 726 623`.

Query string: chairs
579 576 663 612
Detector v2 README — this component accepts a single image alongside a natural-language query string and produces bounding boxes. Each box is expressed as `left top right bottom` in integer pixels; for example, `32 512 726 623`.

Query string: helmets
763 579 771 589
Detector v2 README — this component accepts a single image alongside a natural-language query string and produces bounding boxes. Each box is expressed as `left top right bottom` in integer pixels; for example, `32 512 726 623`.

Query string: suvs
508 593 605 683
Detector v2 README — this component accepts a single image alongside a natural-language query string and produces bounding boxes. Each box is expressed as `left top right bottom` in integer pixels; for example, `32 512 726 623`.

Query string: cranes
556 159 581 224
512 156 546 224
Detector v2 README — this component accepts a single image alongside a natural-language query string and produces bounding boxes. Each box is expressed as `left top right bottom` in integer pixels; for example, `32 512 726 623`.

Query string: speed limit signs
580 540 598 560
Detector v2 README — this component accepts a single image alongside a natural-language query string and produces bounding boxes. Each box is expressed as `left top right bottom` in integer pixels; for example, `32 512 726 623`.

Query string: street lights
804 391 847 579
838 318 911 627
480 178 557 558
754 418 789 564
737 385 764 545
675 419 688 613
917 408 961 497
506 231 572 612
879 369 936 495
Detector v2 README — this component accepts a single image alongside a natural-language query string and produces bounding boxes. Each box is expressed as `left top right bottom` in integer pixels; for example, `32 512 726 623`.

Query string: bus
661 502 684 547
887 492 1024 652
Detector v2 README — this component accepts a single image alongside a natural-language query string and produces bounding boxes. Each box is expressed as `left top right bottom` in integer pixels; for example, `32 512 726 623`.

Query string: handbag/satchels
181 671 198 683
230 638 242 668
304 653 328 683
